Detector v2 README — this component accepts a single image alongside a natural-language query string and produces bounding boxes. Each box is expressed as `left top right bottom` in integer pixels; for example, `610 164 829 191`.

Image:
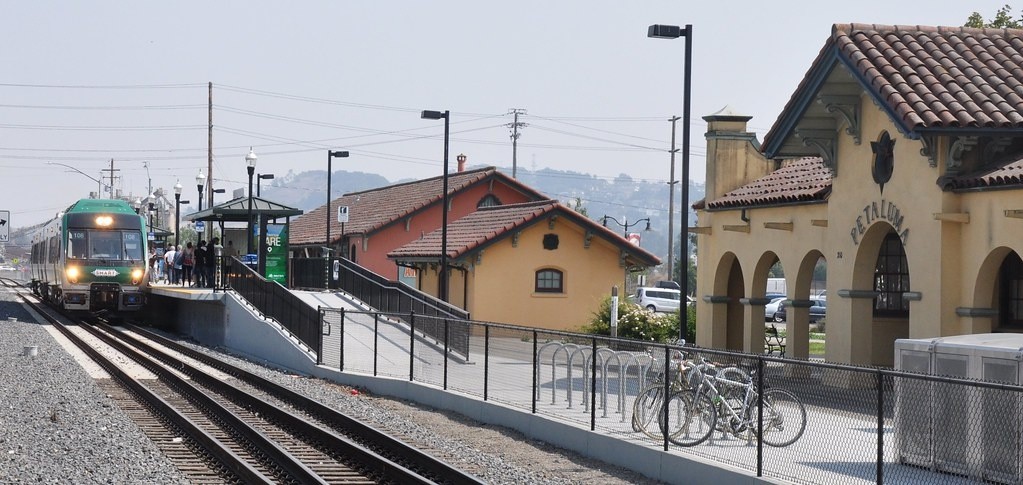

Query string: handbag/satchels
177 249 186 266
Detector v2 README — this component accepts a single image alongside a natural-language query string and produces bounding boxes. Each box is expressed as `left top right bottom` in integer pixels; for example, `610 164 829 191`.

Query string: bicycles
633 337 806 448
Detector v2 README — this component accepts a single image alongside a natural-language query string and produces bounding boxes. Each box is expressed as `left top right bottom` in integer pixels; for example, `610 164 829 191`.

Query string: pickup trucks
638 281 680 300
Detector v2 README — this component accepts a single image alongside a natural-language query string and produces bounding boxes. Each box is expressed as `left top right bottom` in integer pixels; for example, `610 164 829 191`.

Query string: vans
634 287 693 313
810 289 826 302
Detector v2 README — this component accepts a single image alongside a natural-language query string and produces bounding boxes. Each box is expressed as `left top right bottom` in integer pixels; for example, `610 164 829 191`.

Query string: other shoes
163 278 168 284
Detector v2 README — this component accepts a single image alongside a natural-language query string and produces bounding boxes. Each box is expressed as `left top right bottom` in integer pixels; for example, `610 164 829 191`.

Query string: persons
204 243 216 288
172 245 183 285
194 242 206 288
163 260 168 284
201 239 207 263
225 241 237 286
149 249 160 283
180 242 195 286
164 246 176 285
162 243 173 258
214 237 223 286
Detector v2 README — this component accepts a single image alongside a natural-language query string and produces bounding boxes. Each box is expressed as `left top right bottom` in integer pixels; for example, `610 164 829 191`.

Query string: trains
31 199 150 322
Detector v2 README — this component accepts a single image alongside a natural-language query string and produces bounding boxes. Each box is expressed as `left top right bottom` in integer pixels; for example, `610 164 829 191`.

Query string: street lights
196 169 205 245
257 173 274 197
211 188 225 239
135 198 142 214
602 215 654 241
148 191 157 253
327 150 349 249
647 24 692 341
421 109 449 300
44 161 113 198
174 178 191 252
245 146 257 254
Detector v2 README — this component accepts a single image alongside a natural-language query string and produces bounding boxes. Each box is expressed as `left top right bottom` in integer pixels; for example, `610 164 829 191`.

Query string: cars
765 298 787 321
776 299 827 324
